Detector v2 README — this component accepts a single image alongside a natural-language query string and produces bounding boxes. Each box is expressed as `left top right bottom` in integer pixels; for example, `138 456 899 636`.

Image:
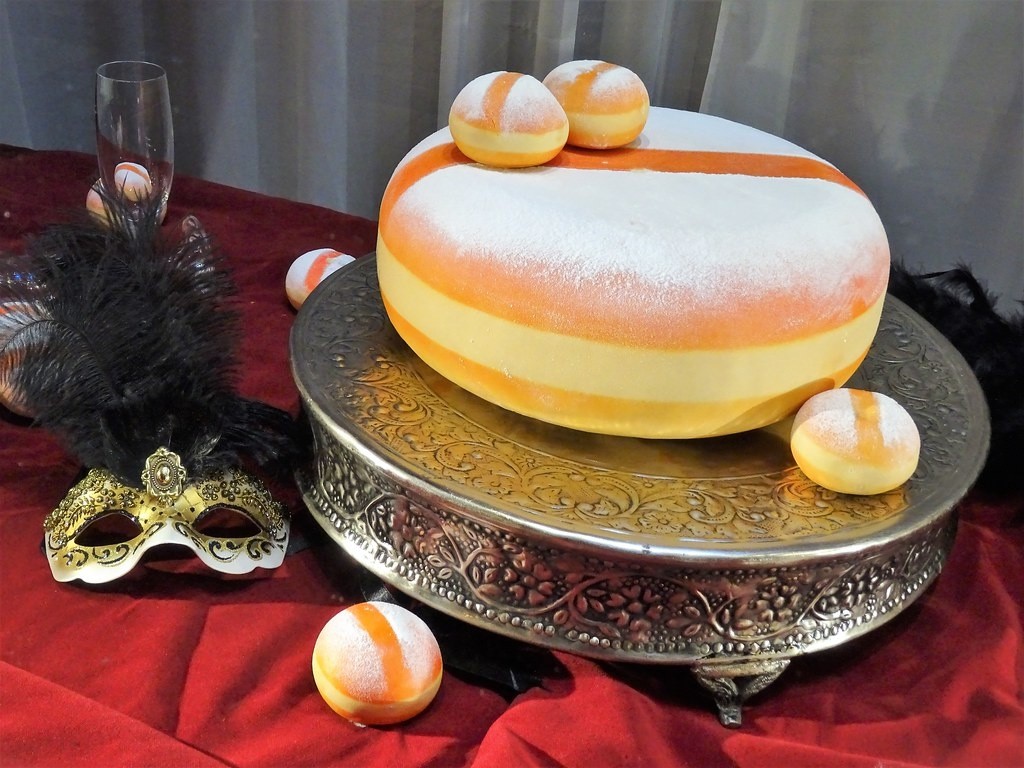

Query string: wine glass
94 61 174 238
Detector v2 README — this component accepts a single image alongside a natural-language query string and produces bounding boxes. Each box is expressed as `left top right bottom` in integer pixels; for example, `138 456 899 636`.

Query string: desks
0 142 1024 768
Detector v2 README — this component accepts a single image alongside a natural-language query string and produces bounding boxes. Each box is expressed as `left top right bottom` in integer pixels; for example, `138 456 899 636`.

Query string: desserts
286 248 357 311
311 601 443 725
790 388 920 496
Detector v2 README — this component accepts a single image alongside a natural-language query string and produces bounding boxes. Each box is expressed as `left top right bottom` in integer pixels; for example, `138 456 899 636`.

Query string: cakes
372 57 890 440
